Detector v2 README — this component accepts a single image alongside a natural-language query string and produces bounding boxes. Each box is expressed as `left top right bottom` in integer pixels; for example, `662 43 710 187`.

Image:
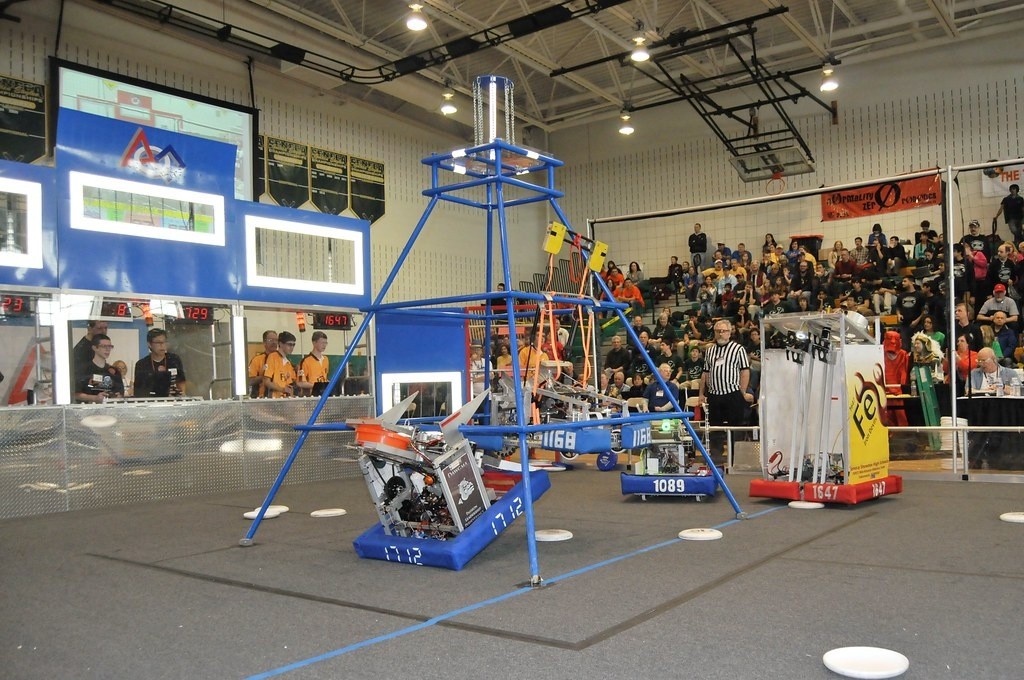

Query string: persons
470 219 1024 441
994 183 1024 244
74 320 108 368
133 328 187 402
74 333 125 404
698 318 752 467
263 332 297 398
247 329 278 398
296 330 330 398
113 360 133 398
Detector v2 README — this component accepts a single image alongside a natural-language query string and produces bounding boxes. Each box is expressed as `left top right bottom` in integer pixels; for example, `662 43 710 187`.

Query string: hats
776 244 783 249
969 220 980 227
470 339 482 348
994 284 1005 293
715 260 722 263
717 241 725 245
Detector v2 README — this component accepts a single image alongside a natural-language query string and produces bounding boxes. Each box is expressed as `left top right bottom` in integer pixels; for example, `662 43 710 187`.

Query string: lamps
617 112 634 134
820 63 840 92
629 31 651 62
406 2 427 31
440 89 457 116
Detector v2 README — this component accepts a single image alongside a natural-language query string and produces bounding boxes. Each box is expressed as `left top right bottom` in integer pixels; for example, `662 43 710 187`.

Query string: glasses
471 348 481 351
97 344 114 350
285 343 295 347
265 338 278 343
714 329 730 333
151 341 168 346
718 244 724 246
975 357 991 364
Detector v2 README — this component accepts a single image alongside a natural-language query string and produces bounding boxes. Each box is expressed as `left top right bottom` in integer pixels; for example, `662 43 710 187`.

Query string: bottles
911 381 917 396
997 378 1004 397
1010 377 1021 396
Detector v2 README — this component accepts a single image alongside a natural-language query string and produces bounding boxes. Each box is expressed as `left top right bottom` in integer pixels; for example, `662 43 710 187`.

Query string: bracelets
955 355 959 358
1003 384 1005 389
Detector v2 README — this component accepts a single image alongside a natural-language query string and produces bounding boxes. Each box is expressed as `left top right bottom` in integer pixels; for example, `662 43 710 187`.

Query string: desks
957 395 1024 471
886 394 923 426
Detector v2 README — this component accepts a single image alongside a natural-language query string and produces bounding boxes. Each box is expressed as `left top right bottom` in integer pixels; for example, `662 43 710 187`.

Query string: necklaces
984 365 998 379
151 353 166 371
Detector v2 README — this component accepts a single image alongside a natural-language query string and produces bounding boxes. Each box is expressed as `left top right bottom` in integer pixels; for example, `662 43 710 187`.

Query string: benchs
566 280 676 365
